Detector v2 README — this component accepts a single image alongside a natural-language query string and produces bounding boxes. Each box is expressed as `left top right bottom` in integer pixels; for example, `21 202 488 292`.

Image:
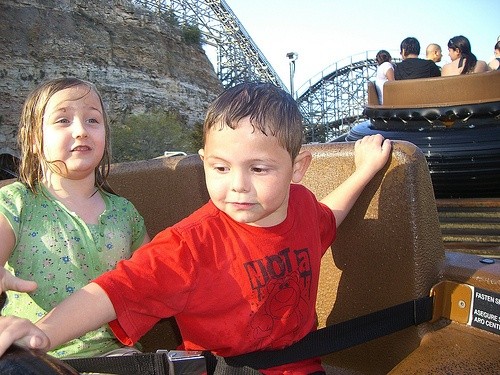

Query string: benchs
0 140 500 375
368 69 500 107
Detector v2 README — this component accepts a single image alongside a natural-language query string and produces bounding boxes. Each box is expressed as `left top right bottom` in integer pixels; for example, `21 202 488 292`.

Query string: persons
484 40 500 71
373 49 395 104
441 34 488 76
0 81 393 375
395 37 441 81
425 43 444 63
0 77 154 354
